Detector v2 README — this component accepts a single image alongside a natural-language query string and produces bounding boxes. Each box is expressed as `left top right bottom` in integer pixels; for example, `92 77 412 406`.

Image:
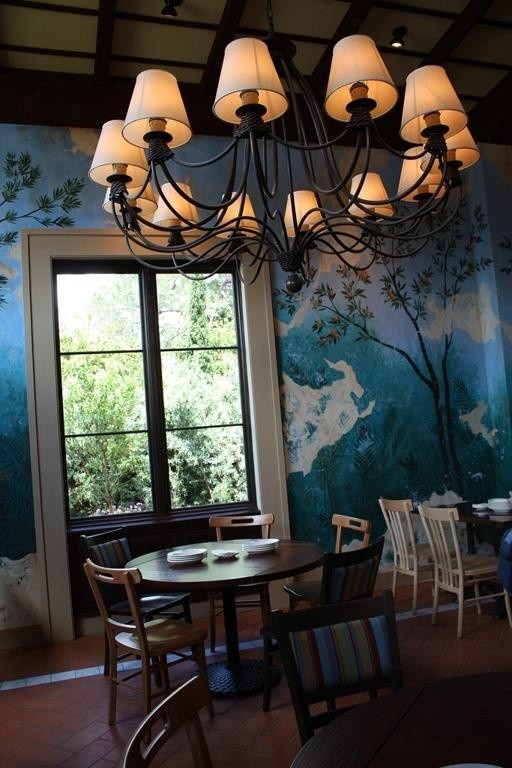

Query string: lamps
88 0 481 293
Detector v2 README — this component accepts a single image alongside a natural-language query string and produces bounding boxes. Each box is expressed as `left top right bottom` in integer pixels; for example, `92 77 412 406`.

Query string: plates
212 551 239 557
167 548 207 566
470 498 512 514
242 538 279 556
